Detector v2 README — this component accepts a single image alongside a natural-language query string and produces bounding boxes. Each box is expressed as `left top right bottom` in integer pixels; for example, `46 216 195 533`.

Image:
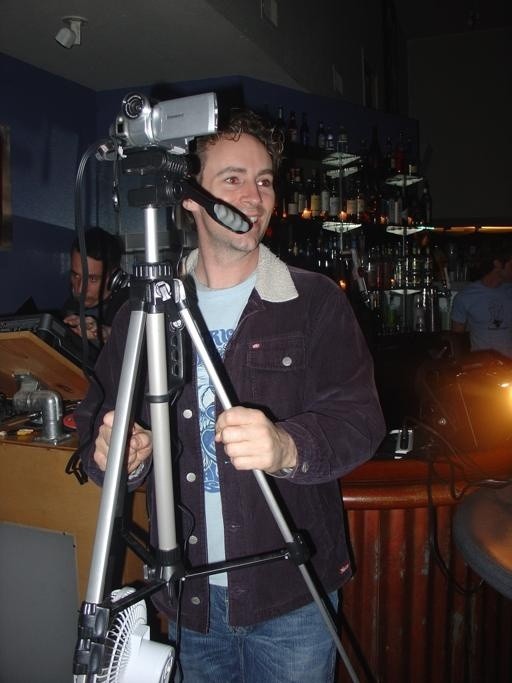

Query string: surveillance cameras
54 27 76 50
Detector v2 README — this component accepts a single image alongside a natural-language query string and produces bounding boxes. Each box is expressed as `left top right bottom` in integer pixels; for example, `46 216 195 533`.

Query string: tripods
69 202 360 683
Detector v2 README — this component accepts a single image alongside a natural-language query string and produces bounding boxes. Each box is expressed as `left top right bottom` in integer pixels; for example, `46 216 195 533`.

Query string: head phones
107 264 125 292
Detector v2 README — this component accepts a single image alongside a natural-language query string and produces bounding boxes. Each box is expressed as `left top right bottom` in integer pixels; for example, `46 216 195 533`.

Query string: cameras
105 85 220 155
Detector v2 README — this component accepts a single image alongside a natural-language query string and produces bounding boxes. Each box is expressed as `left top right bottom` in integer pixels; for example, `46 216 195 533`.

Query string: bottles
256 104 458 335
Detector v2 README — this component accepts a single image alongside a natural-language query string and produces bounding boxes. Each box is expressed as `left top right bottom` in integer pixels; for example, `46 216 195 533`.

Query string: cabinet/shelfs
261 141 431 292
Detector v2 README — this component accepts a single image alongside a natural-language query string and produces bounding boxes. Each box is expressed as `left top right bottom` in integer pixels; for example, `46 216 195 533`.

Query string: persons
450 236 512 361
61 227 129 350
75 105 388 683
